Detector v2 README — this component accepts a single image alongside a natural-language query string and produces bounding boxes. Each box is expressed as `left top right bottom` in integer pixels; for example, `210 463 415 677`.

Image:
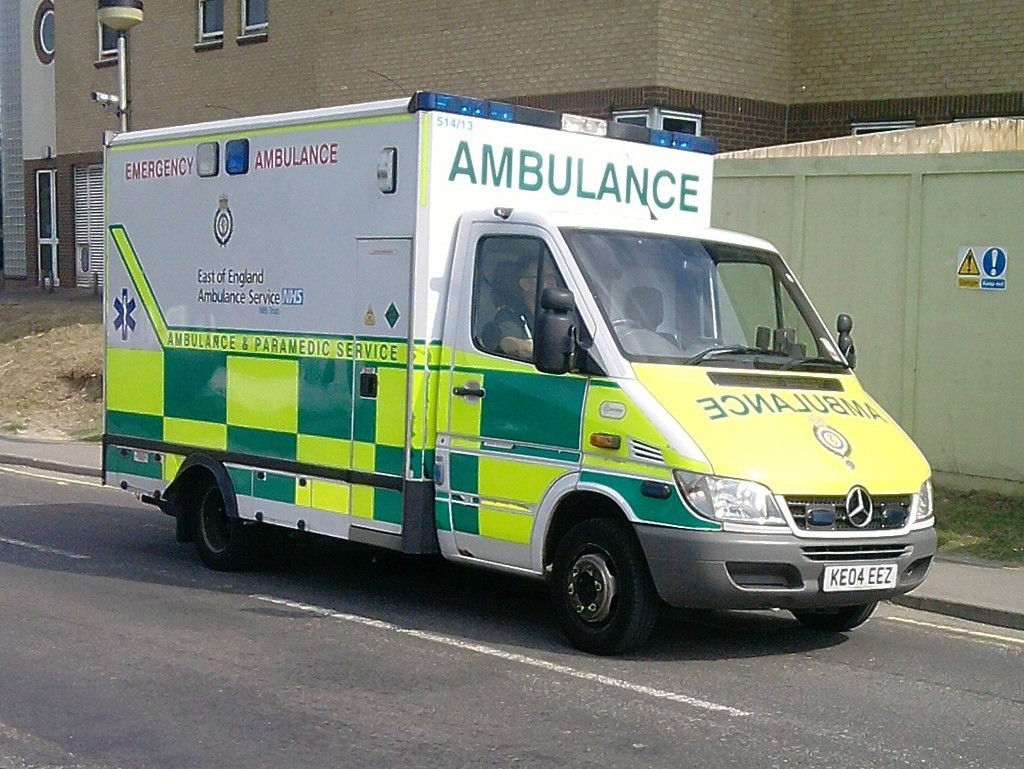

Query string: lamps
40 145 51 159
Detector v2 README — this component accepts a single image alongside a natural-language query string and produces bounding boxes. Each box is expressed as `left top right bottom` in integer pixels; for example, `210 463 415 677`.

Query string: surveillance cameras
91 91 120 105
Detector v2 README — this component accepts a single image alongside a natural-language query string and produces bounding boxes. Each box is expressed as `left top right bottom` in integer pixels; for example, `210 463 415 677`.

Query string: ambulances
100 90 940 659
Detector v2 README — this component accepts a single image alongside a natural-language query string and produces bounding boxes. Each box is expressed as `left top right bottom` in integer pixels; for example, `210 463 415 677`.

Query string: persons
492 256 558 358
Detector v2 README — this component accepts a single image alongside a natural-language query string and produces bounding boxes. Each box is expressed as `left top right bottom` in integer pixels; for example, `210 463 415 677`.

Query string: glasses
520 274 557 283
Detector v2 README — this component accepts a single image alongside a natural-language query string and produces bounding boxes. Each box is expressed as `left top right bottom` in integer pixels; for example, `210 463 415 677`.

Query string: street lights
89 0 144 132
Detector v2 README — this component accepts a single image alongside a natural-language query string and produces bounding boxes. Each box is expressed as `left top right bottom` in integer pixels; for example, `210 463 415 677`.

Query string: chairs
481 262 528 355
622 286 681 352
